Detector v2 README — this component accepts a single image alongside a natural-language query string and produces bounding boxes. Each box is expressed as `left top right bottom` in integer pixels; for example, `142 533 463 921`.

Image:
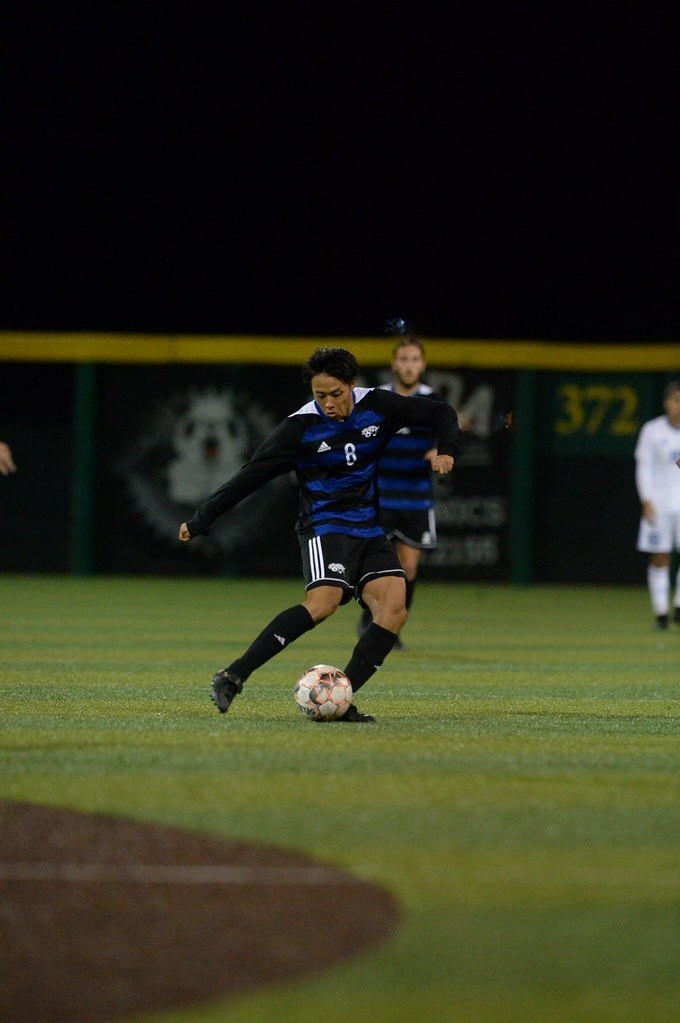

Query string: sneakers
336 705 374 724
210 667 244 713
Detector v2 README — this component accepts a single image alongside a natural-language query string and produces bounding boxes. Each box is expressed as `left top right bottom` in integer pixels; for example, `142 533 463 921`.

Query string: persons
178 349 458 727
356 341 452 650
634 381 680 630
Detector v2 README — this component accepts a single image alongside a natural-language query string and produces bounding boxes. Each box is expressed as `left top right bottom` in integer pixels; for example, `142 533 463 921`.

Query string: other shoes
673 610 680 622
658 616 667 629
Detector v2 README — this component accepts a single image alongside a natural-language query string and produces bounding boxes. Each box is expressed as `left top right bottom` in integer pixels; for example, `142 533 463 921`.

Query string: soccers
293 663 353 722
